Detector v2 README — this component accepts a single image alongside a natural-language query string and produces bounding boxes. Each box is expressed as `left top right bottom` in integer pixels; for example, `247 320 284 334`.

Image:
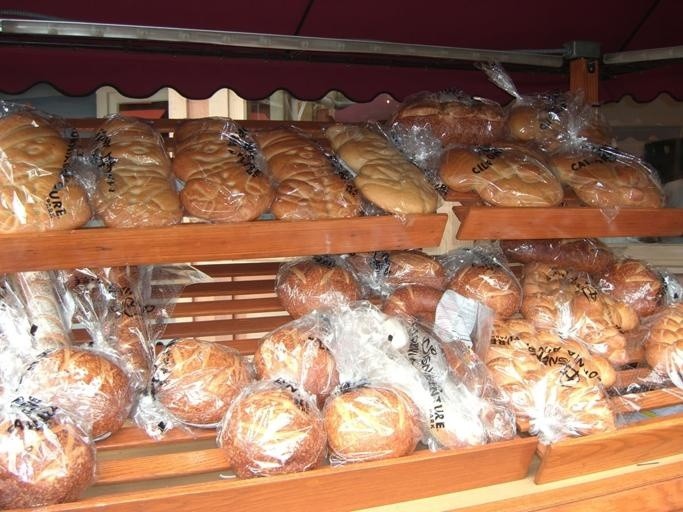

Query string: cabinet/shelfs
0 116 682 510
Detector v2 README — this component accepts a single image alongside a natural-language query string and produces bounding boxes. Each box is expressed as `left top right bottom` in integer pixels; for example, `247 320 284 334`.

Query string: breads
220 383 328 478
439 139 565 207
91 113 184 229
325 120 440 217
251 126 364 222
392 92 506 150
153 336 258 426
0 262 152 512
253 322 347 410
276 236 683 435
0 109 92 232
547 134 666 209
508 101 612 152
319 384 423 463
172 115 276 222
